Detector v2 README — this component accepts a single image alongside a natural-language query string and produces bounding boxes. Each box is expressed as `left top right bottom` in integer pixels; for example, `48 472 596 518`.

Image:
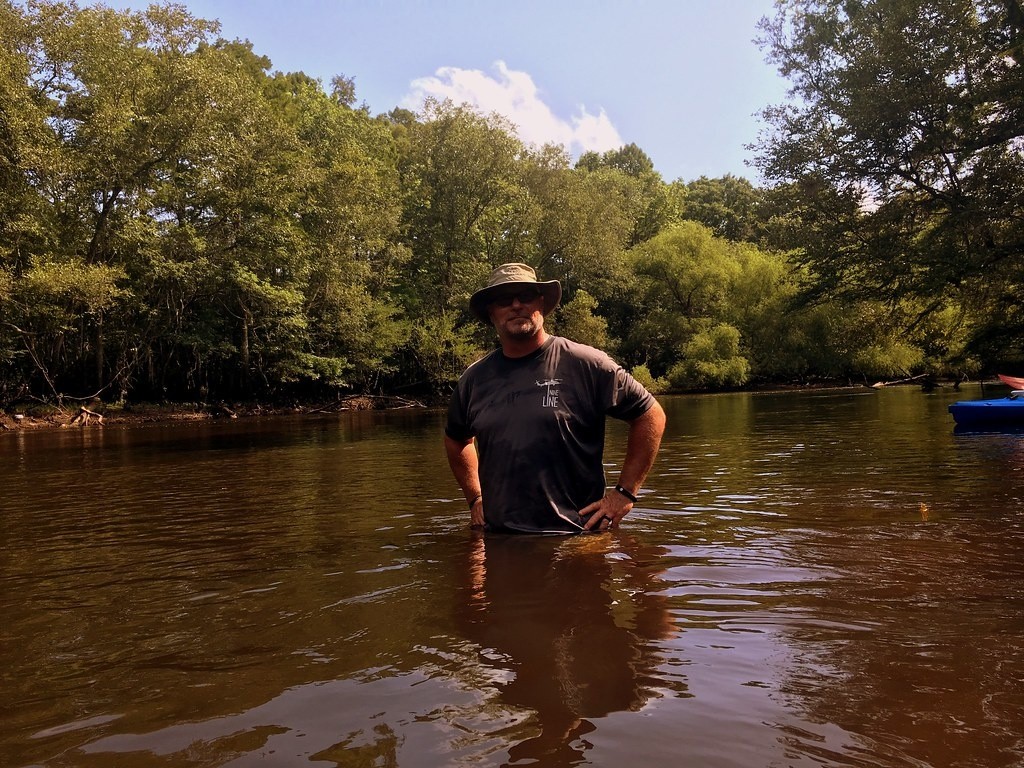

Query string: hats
469 263 562 327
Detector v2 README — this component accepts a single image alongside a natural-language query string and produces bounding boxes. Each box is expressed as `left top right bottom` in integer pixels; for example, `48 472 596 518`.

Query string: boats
997 375 1024 389
947 399 1024 425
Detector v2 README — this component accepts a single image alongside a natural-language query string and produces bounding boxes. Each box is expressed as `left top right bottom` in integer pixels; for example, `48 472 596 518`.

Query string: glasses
490 288 543 307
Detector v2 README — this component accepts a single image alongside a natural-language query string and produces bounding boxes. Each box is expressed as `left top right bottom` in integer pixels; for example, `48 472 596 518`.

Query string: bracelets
614 483 638 503
468 495 482 509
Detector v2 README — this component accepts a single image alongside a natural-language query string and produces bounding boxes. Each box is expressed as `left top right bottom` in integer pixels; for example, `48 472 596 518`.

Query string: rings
604 515 612 523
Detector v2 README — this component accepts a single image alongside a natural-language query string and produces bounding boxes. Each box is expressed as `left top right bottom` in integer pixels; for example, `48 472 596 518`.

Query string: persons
440 260 668 535
